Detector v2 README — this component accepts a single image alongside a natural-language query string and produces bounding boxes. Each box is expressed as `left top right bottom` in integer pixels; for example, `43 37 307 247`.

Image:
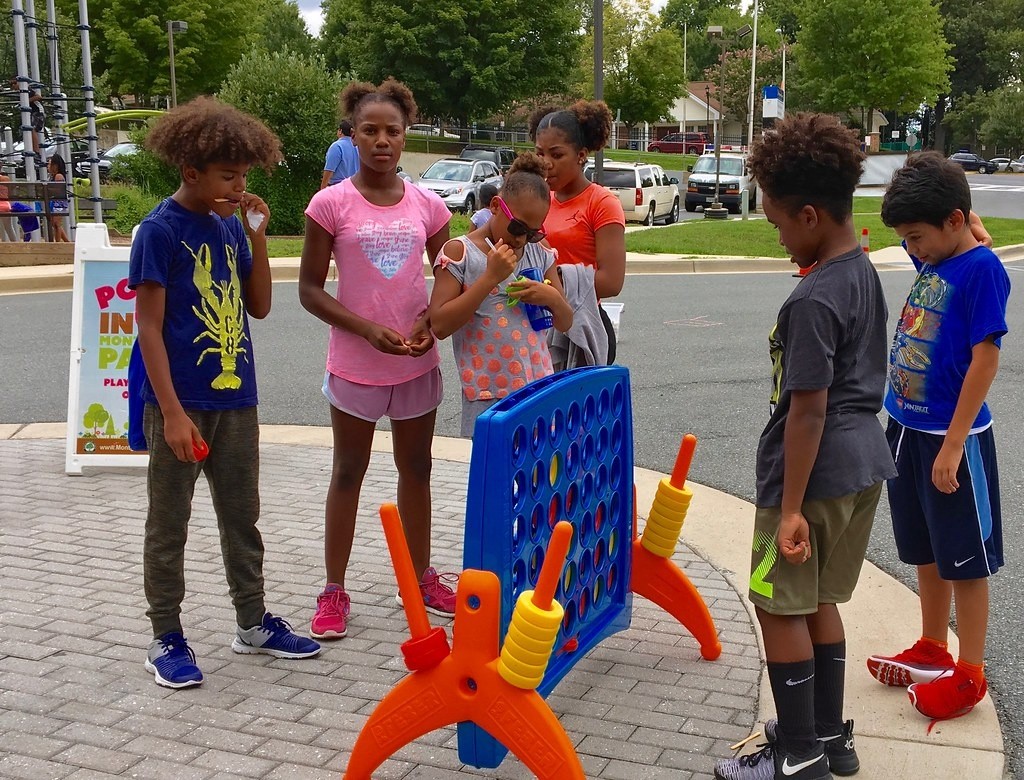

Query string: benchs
0 182 69 242
75 198 117 223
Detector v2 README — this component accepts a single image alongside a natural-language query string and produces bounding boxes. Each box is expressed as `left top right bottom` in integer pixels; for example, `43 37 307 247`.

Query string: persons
711 112 899 780
864 149 1010 723
0 154 70 242
526 100 626 372
428 151 555 438
126 94 322 691
299 78 456 638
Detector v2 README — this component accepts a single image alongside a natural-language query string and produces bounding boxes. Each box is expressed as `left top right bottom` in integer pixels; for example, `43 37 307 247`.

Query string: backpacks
116 214 243 453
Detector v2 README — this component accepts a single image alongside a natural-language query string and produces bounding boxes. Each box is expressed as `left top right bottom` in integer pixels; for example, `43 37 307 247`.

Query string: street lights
167 18 188 111
704 23 752 220
775 28 786 119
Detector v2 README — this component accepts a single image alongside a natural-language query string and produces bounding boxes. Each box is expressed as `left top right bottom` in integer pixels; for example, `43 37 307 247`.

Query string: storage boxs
600 302 626 343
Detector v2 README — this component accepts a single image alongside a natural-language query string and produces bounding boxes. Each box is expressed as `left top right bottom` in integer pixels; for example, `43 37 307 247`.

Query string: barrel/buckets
599 303 625 343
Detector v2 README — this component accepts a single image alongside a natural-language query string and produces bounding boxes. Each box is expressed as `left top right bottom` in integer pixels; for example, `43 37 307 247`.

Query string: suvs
685 143 757 215
647 132 711 156
946 153 1000 174
458 143 520 182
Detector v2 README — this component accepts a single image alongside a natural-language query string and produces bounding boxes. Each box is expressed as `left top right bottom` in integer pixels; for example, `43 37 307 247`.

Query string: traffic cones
859 229 870 258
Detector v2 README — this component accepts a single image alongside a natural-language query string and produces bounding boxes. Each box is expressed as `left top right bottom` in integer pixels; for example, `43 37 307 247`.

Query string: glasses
498 196 547 243
336 128 342 130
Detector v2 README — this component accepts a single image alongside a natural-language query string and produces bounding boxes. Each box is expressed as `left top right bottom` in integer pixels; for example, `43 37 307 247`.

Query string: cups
517 267 554 331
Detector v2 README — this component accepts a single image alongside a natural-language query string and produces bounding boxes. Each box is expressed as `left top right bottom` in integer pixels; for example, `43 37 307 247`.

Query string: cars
989 158 1024 173
405 124 460 139
582 157 613 172
0 128 74 183
1018 155 1024 163
584 162 681 227
75 142 147 184
414 157 506 218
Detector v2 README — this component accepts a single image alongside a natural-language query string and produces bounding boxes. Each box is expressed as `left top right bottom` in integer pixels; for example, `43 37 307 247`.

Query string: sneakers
867 639 957 687
231 612 323 659
765 718 860 776
145 631 203 688
714 724 833 780
395 567 460 618
907 665 987 736
309 583 350 638
